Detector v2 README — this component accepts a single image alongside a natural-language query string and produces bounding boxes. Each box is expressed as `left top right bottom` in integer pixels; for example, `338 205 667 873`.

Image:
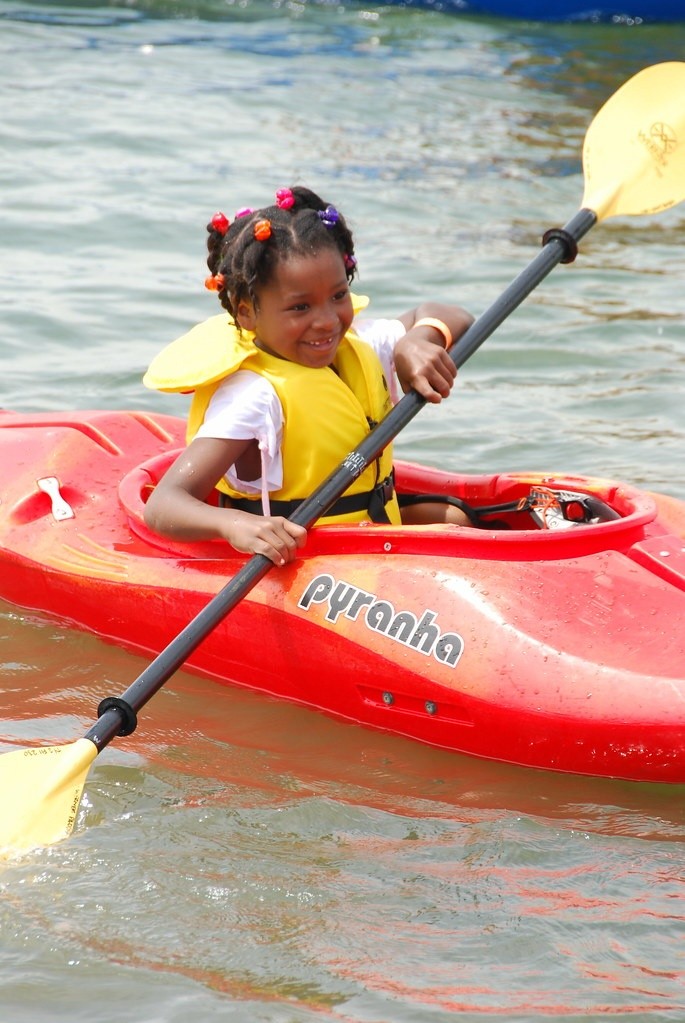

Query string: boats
0 409 685 782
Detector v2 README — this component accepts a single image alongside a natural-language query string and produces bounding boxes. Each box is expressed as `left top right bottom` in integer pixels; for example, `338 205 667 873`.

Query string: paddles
0 60 684 838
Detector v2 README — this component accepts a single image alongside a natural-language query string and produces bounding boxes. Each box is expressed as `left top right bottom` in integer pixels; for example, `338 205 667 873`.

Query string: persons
143 187 474 567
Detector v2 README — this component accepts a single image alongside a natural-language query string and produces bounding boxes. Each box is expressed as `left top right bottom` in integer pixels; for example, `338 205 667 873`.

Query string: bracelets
412 318 452 351
